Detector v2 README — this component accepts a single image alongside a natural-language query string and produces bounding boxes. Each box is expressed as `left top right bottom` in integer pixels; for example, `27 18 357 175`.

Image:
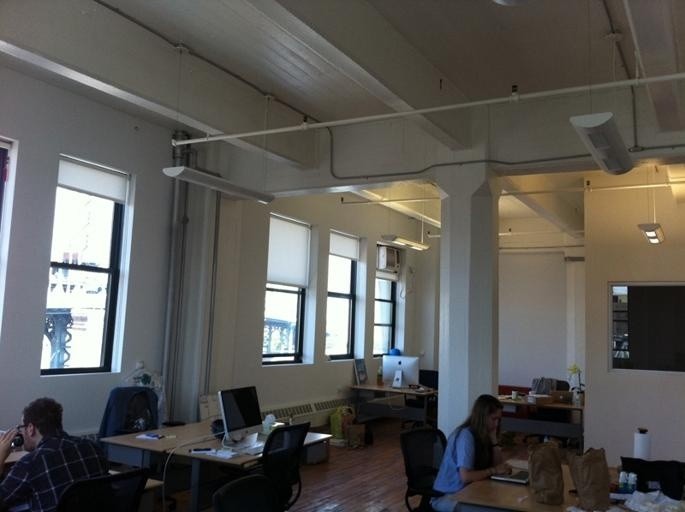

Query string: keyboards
245 446 265 455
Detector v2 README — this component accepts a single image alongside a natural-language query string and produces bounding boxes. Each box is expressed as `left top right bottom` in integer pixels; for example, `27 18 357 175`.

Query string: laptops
552 391 574 403
490 466 530 484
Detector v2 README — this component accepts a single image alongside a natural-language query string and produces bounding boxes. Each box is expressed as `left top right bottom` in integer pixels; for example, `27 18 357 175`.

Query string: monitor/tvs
383 355 420 389
218 385 264 448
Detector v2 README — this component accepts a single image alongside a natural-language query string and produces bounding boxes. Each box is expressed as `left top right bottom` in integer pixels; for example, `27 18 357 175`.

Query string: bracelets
490 441 500 448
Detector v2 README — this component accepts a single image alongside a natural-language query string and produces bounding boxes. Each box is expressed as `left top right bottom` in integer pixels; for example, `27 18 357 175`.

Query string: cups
512 390 518 400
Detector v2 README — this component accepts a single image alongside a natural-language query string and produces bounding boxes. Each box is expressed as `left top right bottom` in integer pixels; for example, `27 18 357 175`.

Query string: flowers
568 363 585 393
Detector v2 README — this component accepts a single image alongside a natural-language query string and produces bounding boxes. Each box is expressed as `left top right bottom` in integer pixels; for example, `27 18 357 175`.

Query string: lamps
379 182 430 251
568 0 636 175
638 160 665 244
161 43 274 207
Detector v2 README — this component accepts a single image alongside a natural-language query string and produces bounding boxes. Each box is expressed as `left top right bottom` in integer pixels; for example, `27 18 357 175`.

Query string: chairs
55 467 148 512
102 388 187 511
523 379 569 448
401 370 438 431
400 430 447 512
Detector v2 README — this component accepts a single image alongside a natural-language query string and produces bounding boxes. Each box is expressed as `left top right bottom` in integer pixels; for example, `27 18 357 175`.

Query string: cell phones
193 448 211 452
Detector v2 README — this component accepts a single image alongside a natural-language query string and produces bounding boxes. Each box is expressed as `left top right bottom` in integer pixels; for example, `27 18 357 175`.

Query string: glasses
17 424 28 432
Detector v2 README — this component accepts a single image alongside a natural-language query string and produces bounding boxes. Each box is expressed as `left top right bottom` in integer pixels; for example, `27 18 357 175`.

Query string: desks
101 417 333 511
350 383 436 429
448 459 624 512
498 395 583 456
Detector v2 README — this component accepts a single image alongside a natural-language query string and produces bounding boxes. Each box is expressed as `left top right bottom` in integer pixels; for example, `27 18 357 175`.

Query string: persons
0 396 111 512
428 394 511 512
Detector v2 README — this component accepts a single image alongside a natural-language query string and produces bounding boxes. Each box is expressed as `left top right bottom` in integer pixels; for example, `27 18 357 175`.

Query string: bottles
377 366 384 386
575 390 581 407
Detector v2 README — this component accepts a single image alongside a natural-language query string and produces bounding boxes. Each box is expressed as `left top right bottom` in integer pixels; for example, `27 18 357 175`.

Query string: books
206 449 239 459
135 431 162 439
490 458 529 484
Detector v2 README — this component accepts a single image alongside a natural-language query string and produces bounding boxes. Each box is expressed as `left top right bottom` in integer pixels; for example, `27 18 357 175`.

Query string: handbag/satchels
330 406 368 450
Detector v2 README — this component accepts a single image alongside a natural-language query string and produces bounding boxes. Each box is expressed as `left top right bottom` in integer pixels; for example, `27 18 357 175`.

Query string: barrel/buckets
330 406 356 438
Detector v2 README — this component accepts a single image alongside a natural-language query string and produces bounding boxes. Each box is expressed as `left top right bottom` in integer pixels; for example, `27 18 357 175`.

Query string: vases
573 394 585 407
